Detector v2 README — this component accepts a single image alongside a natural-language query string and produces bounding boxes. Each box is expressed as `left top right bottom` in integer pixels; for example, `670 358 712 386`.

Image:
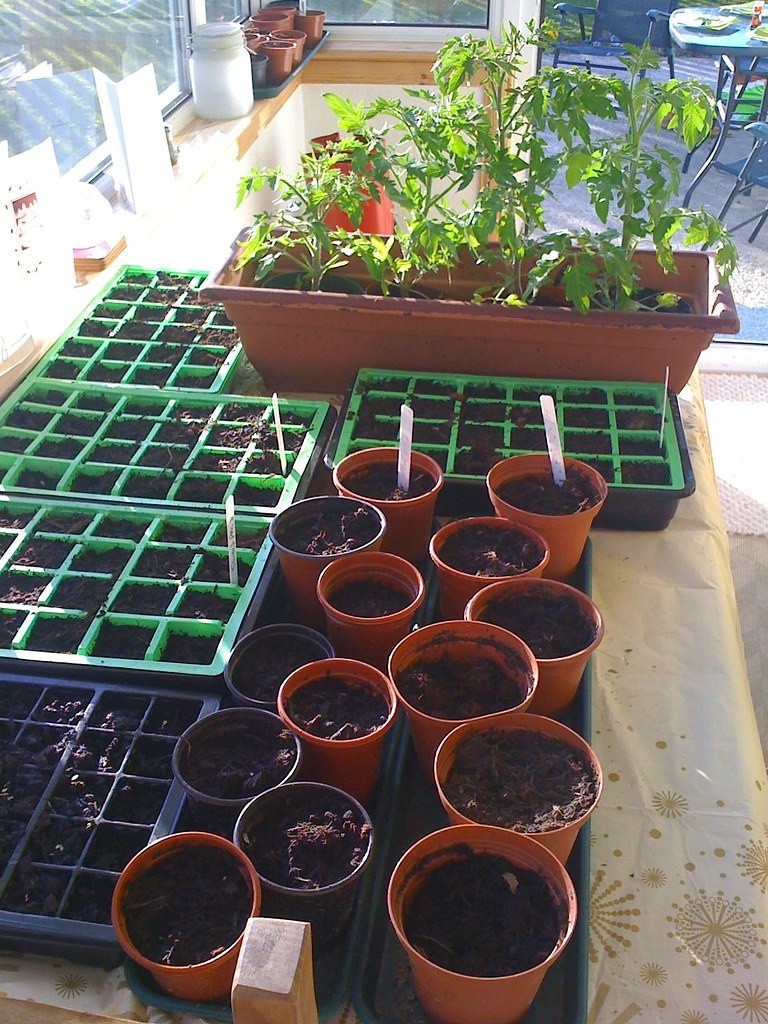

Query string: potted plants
198 18 739 393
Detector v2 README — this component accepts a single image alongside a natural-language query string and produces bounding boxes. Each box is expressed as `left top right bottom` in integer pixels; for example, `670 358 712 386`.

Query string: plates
678 11 737 30
746 25 768 43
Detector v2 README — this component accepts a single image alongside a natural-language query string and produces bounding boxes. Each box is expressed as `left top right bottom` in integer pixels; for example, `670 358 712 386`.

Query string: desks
669 3 768 174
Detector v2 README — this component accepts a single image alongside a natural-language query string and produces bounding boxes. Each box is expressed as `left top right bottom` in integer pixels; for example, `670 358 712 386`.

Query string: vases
434 713 602 866
487 454 608 581
269 496 387 630
462 579 604 719
0 671 224 968
430 516 551 620
0 494 282 706
111 834 261 1006
243 0 325 89
316 551 427 676
28 263 244 393
324 367 696 529
224 623 335 713
234 781 376 955
169 707 303 841
332 447 445 566
386 620 539 790
387 825 576 1024
275 659 397 804
0 379 338 517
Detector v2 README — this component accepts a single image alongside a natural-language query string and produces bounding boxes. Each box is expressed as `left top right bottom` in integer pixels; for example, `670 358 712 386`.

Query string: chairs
552 0 768 244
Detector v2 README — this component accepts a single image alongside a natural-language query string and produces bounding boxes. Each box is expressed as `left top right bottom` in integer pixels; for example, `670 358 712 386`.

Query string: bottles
750 0 765 30
